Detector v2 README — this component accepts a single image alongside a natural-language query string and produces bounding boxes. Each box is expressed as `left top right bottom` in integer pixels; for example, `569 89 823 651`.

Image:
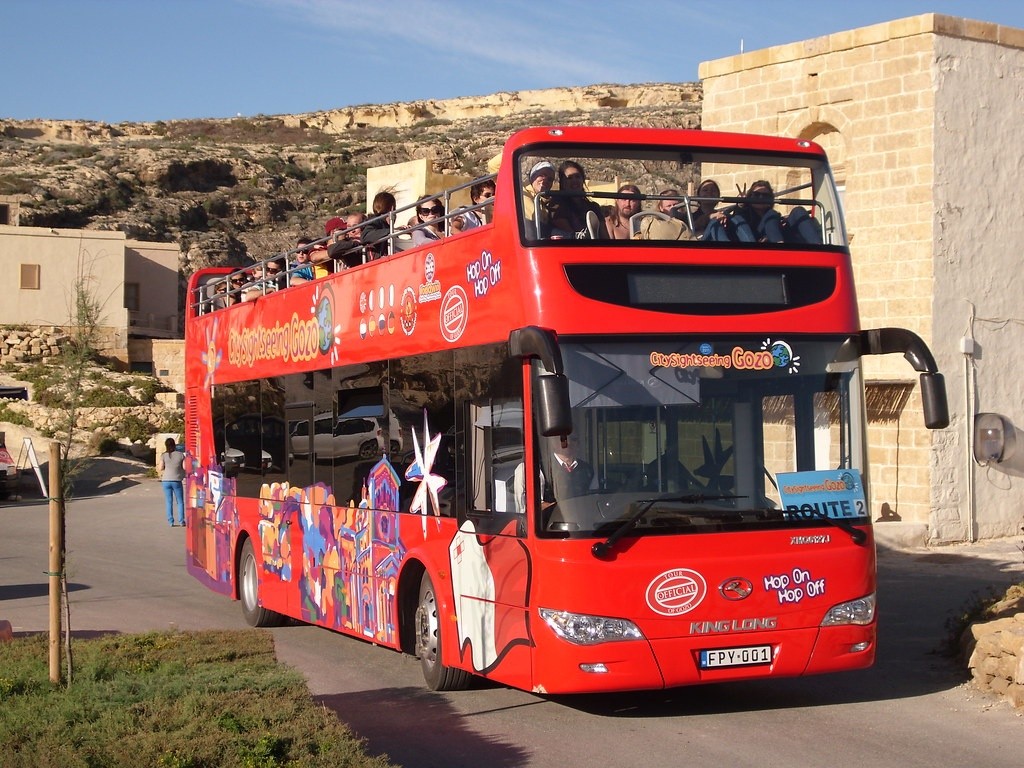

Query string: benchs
599 464 668 494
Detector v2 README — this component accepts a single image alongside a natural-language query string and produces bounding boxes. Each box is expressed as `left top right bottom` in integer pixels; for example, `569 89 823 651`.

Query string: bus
185 118 949 696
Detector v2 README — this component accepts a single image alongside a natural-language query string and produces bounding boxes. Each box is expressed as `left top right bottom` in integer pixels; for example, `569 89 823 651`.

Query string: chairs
337 210 699 274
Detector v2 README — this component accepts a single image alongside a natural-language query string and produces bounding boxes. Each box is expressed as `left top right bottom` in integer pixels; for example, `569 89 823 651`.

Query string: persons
160 438 187 527
525 422 599 513
205 161 824 311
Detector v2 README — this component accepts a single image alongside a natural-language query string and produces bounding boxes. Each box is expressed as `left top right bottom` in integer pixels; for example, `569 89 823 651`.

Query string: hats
529 161 555 184
325 217 347 234
696 178 721 198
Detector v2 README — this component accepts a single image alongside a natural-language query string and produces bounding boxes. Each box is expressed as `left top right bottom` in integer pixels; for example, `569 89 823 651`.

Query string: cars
402 401 525 489
225 444 244 474
263 450 273 470
0 439 19 502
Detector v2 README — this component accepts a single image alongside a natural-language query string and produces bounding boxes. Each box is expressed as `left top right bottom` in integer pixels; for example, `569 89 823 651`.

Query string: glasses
420 206 438 216
266 267 277 274
218 287 227 292
231 277 245 283
295 247 309 254
481 192 495 198
750 191 770 198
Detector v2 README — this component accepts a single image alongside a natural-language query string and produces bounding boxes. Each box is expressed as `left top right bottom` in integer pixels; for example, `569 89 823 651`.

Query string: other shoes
170 523 174 527
577 211 599 239
180 523 186 527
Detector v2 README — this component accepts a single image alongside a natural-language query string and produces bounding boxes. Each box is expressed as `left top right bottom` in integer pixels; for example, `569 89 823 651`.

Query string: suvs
291 411 402 461
